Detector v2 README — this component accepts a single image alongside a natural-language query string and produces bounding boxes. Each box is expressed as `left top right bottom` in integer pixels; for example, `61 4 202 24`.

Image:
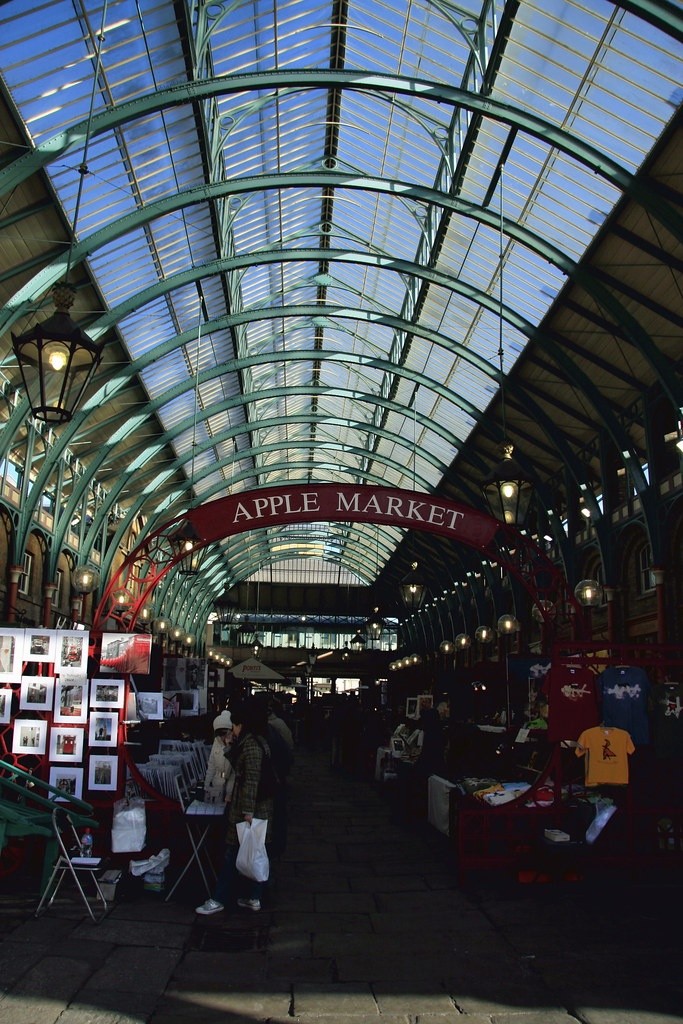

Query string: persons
23 737 35 746
58 780 70 794
99 725 107 740
195 700 294 914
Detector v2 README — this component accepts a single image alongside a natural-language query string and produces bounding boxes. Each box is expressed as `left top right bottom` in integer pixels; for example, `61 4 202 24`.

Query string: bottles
80 828 92 858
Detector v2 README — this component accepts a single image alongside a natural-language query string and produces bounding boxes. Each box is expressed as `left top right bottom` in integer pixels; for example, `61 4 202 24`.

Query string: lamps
399 389 426 615
475 160 539 532
533 601 556 623
389 654 424 672
10 0 112 424
572 579 603 610
340 610 386 660
76 297 265 668
439 614 519 656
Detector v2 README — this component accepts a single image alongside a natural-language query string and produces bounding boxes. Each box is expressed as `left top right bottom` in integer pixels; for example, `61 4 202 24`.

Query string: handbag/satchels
235 818 269 881
111 799 146 853
129 848 171 877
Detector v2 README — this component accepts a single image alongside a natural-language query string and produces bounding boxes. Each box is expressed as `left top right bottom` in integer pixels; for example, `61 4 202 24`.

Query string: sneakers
237 898 261 911
195 899 225 915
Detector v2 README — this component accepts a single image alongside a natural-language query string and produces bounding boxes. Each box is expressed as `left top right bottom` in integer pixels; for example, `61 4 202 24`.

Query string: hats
213 709 232 731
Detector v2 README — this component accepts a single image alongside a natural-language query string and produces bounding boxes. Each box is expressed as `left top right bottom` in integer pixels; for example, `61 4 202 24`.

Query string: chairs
34 807 109 922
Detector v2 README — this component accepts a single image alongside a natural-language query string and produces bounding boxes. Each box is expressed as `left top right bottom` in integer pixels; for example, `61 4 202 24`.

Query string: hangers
662 676 678 686
614 657 631 672
598 719 612 733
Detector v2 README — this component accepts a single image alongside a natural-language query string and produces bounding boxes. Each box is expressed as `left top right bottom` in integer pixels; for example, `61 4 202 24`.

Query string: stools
164 773 219 902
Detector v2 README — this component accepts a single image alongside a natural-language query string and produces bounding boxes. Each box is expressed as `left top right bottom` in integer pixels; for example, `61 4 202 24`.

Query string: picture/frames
0 627 210 803
